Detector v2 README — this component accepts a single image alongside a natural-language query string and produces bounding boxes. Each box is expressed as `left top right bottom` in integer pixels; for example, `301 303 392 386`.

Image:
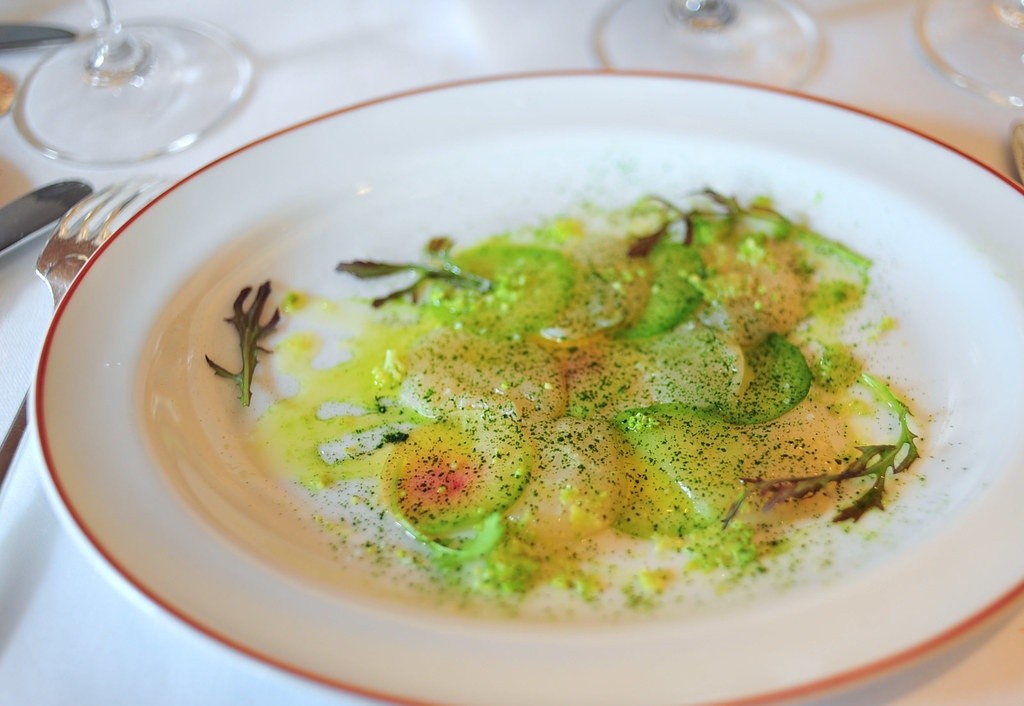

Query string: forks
0 172 174 498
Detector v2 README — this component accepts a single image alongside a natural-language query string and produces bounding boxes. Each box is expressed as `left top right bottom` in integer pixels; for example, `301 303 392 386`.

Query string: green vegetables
197 190 921 521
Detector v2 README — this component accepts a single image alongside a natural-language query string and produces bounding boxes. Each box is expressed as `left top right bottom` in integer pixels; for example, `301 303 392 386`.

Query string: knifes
0 24 76 51
0 177 90 255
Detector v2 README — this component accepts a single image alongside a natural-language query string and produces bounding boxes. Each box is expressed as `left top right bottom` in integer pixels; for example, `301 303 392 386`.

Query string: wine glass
597 0 827 93
917 1 1024 104
14 0 253 165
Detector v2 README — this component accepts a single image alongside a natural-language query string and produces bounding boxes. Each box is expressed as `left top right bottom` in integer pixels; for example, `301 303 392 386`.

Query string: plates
28 69 1024 706
